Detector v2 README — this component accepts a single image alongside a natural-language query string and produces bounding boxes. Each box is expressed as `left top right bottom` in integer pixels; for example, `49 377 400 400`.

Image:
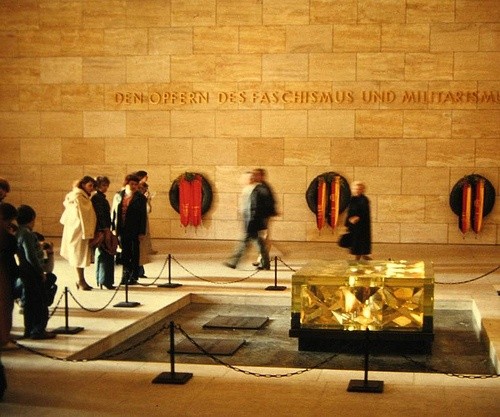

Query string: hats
247 169 264 174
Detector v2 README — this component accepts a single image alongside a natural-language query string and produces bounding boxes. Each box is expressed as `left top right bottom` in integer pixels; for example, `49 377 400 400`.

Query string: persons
0 177 58 351
59 170 155 291
222 169 281 271
342 181 374 261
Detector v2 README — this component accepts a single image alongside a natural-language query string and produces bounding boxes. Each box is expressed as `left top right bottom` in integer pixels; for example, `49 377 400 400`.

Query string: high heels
97 282 116 289
76 281 93 290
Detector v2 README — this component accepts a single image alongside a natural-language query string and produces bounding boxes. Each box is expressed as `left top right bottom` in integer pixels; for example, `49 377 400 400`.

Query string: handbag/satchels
98 230 118 256
338 232 352 248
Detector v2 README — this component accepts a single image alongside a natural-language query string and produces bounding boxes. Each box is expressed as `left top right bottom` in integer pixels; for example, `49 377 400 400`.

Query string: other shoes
139 274 147 278
138 235 151 266
149 251 158 254
0 341 22 352
120 279 137 285
24 325 57 339
253 262 259 266
223 262 235 269
258 265 270 270
